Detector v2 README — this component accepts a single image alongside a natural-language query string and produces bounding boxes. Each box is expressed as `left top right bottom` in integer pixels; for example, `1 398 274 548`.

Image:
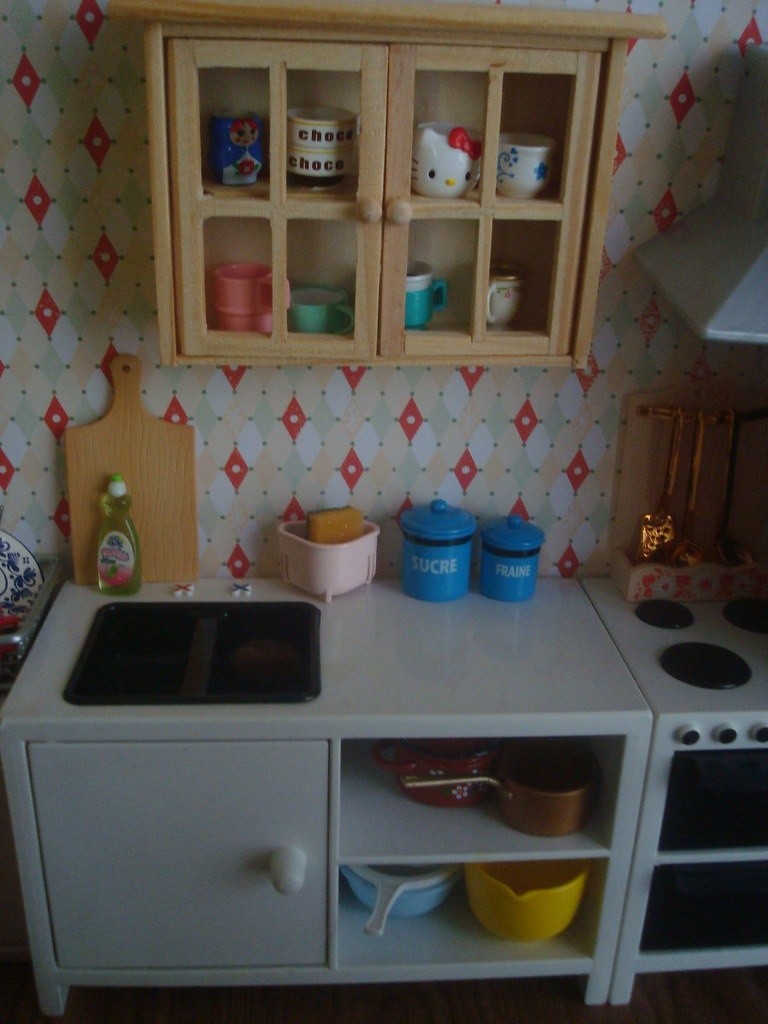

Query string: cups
286 106 358 188
213 263 272 333
287 282 354 334
405 259 448 330
485 263 523 330
476 513 545 603
398 499 477 602
208 109 265 185
410 121 484 198
496 131 562 199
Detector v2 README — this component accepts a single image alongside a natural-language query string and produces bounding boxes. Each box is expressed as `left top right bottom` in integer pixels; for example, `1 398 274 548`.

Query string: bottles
96 473 143 596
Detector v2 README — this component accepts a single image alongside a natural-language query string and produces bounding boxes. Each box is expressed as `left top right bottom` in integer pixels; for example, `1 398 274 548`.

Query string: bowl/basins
337 865 460 918
370 739 505 808
462 859 592 943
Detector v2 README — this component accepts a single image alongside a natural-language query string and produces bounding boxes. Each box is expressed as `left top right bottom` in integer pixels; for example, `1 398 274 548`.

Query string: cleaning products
95 470 144 599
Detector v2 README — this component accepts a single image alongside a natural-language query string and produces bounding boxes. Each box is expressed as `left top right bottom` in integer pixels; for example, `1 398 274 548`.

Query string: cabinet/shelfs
0 577 652 1014
108 0 668 367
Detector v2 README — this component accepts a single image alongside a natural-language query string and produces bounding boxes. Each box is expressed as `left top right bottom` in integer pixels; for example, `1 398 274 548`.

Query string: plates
0 529 45 622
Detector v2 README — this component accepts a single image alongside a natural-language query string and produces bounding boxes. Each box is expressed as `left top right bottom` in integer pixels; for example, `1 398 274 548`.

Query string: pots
399 757 604 837
